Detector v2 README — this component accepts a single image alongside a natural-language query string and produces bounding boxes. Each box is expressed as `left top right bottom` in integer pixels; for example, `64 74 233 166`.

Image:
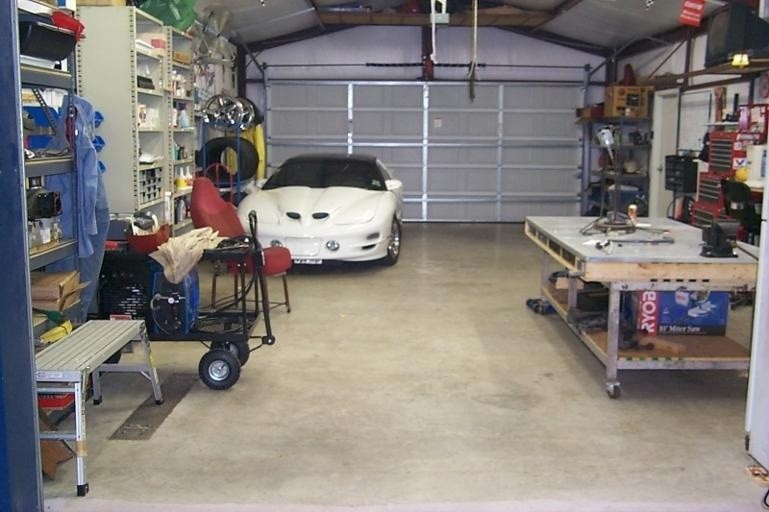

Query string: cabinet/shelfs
17 0 239 341
584 118 652 216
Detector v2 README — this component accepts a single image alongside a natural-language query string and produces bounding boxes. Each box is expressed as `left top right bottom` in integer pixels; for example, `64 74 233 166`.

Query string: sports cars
232 151 406 270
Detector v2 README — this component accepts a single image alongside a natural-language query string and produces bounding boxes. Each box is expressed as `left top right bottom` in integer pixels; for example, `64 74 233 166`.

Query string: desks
524 216 760 399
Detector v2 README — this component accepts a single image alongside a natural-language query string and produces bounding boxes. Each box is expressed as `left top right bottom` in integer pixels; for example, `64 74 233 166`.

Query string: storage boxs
630 290 729 337
604 86 650 119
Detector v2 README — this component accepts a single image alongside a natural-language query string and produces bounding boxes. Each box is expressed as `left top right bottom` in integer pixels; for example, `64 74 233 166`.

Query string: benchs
35 319 166 496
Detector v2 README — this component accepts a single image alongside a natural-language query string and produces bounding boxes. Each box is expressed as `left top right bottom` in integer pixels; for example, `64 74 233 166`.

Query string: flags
677 0 706 28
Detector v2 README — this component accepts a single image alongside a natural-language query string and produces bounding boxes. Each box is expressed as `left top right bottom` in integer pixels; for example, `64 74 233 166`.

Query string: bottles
171 101 189 129
177 166 193 191
172 70 187 98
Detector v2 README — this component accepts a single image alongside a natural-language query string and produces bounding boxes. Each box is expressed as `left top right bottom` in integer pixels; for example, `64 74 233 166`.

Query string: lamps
732 54 749 68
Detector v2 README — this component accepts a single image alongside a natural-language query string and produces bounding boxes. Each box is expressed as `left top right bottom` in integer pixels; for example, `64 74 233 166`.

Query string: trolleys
87 207 276 393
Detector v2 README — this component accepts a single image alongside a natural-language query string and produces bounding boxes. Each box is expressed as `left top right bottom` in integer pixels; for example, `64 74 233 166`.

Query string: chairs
192 176 293 312
721 179 761 244
